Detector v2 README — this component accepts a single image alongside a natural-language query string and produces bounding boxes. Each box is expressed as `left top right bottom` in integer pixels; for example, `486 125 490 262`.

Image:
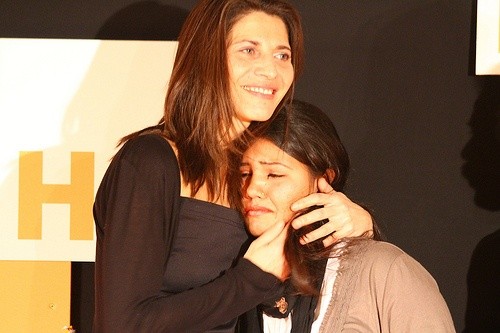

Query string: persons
91 0 375 332
238 99 455 332
